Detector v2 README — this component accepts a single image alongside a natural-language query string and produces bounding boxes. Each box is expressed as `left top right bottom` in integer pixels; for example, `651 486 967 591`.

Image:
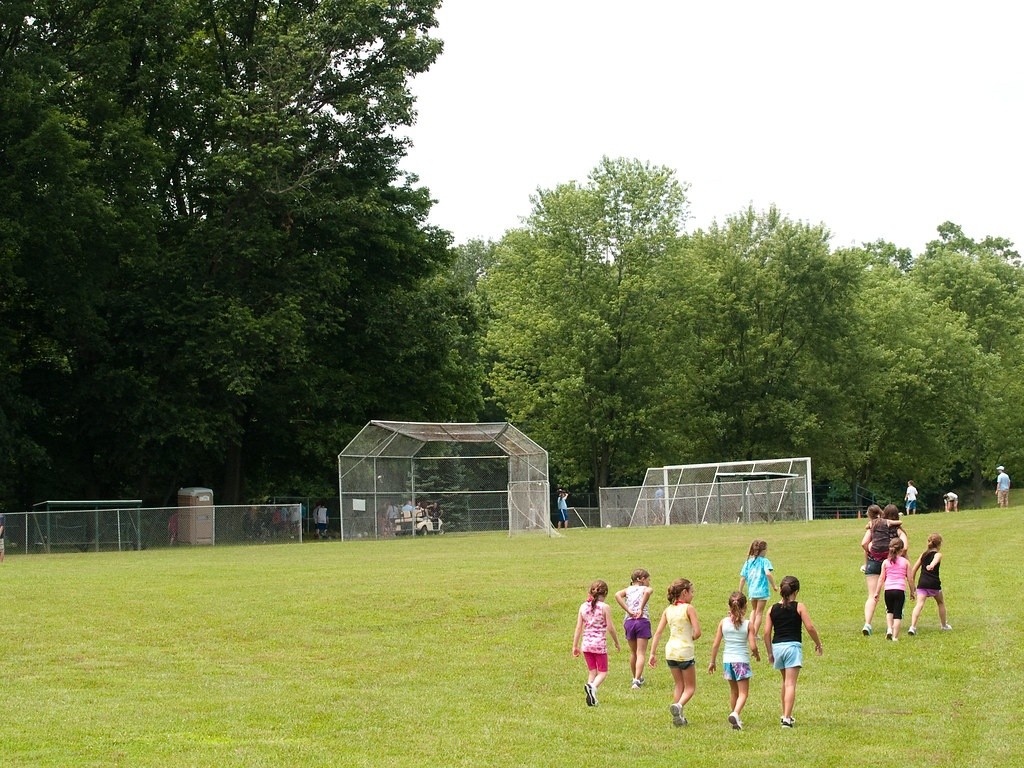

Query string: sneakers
632 676 645 684
632 681 641 689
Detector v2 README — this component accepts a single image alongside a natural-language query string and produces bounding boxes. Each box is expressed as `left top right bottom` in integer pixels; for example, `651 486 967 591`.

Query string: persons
908 533 952 635
763 576 823 728
994 466 1010 508
615 568 653 689
708 591 760 730
557 489 569 529
168 511 178 546
875 538 914 642
943 492 958 512
739 540 777 641
649 578 701 727
242 501 306 542
378 498 443 531
654 484 663 510
904 480 918 515
0 514 5 562
861 503 908 636
572 580 620 706
312 502 330 538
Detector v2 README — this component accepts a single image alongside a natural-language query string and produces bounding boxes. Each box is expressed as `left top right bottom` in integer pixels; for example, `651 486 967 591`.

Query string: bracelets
815 644 822 649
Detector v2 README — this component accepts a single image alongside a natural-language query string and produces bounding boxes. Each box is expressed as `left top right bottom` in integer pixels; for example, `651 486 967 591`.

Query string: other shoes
941 624 952 630
908 626 916 635
863 625 872 636
781 716 795 724
728 712 743 730
893 638 898 641
885 630 892 641
585 683 597 707
782 720 793 728
860 566 865 573
669 703 686 726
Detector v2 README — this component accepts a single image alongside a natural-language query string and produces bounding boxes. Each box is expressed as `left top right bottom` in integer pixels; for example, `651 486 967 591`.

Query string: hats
997 466 1004 470
